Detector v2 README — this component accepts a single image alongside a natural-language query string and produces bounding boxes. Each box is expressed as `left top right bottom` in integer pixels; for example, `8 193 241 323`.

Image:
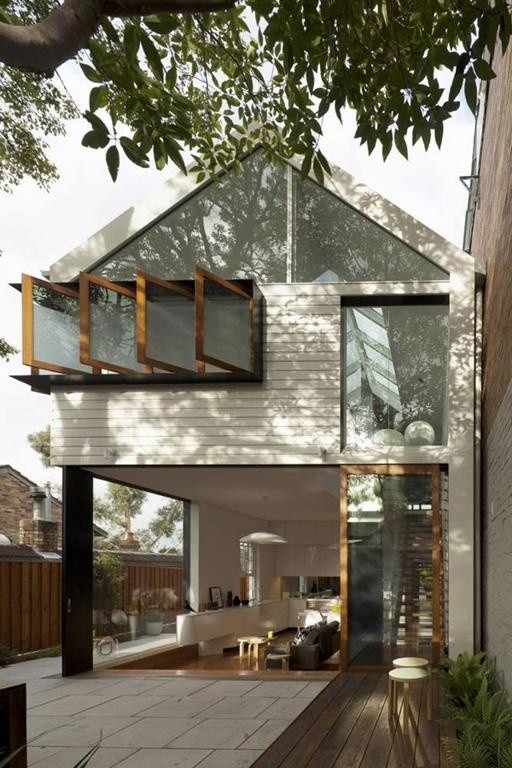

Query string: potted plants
145 609 163 634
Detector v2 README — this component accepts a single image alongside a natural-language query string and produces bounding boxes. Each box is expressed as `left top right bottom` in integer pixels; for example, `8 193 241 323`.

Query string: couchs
267 620 340 671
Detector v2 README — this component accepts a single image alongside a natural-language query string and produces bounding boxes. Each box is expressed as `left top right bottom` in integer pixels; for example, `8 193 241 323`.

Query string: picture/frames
209 586 224 609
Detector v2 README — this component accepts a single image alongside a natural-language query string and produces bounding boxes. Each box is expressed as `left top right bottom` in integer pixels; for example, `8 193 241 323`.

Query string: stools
386 657 434 736
237 636 267 672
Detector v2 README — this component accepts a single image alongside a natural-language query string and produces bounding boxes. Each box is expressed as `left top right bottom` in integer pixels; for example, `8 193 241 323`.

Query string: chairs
296 607 341 636
